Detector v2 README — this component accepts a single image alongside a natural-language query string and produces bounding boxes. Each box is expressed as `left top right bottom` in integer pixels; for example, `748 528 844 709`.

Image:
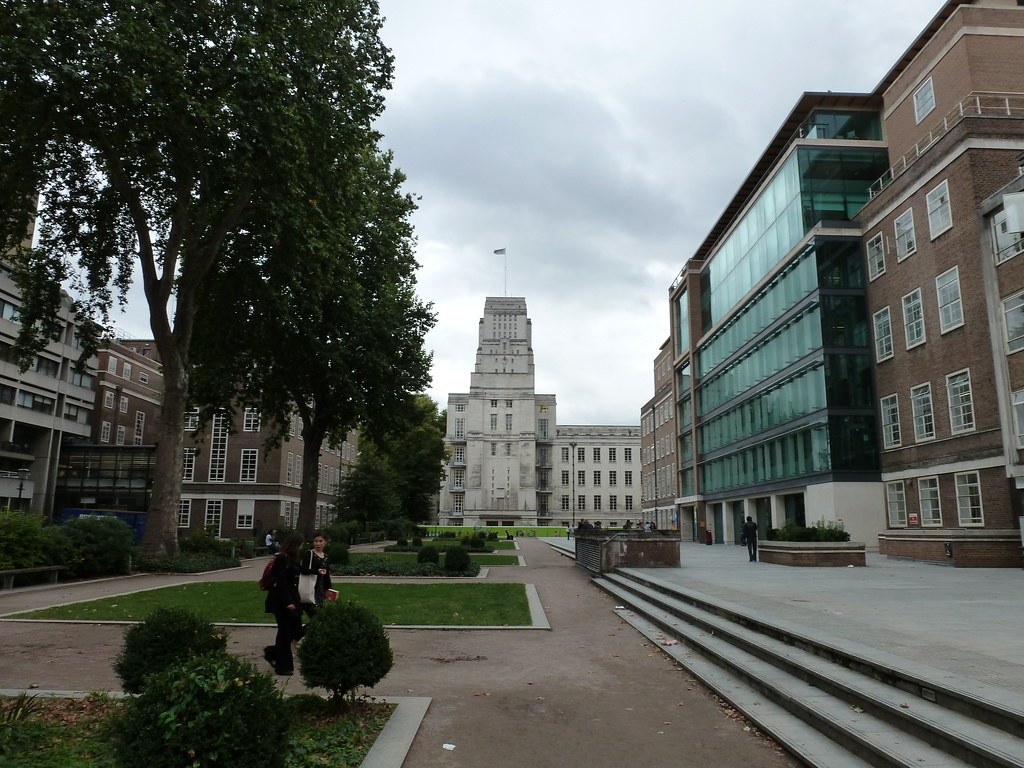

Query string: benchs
504 529 514 540
0 565 69 589
255 547 270 557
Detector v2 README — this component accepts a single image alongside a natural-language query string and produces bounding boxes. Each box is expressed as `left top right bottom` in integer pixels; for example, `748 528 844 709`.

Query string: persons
566 518 657 540
258 528 332 676
743 516 757 562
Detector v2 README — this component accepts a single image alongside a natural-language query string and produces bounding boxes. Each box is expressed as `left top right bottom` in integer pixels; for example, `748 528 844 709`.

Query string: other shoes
264 652 277 668
276 669 294 676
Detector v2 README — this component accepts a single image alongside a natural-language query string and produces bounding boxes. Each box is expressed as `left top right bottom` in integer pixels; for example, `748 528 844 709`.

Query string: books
327 589 339 601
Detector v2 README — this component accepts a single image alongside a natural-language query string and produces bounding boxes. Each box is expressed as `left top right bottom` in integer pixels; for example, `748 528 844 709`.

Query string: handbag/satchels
298 549 318 605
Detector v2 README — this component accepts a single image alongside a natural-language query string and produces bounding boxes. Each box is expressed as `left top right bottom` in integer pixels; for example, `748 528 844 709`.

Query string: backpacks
259 552 295 592
